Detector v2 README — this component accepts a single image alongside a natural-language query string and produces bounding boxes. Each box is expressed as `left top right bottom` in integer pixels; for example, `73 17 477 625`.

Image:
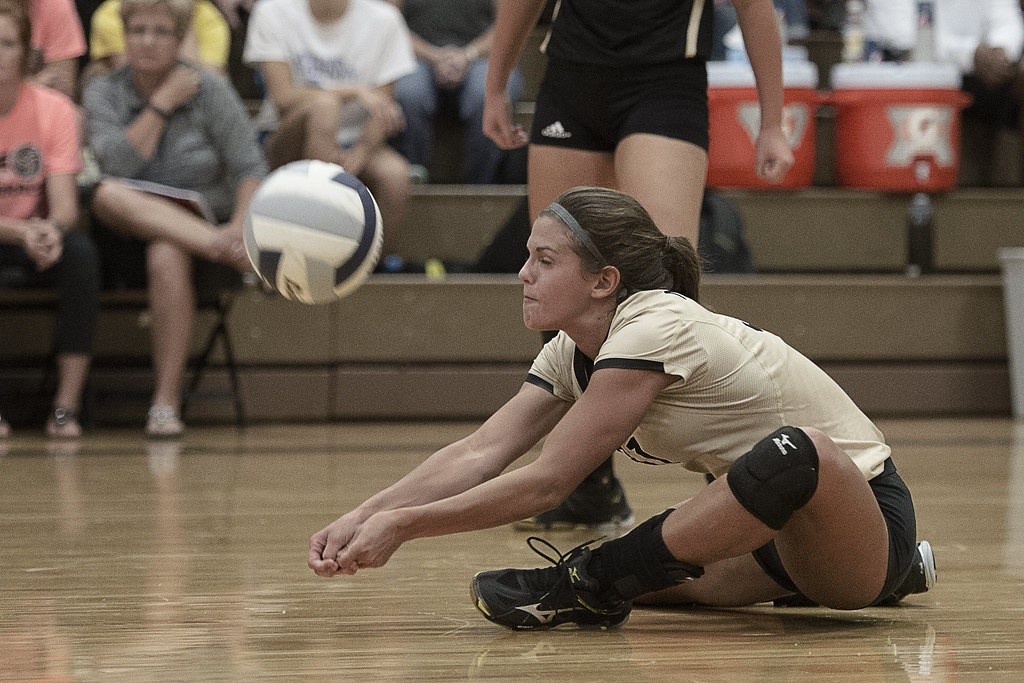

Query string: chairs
0 67 263 426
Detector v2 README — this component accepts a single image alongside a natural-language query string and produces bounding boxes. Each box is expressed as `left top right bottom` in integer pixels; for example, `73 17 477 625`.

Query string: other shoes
143 407 185 439
46 405 83 440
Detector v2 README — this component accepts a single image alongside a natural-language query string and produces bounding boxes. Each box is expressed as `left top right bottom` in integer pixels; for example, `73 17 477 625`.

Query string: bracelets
143 101 173 120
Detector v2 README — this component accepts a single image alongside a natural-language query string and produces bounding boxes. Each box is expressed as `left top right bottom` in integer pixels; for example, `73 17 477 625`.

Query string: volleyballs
243 159 384 307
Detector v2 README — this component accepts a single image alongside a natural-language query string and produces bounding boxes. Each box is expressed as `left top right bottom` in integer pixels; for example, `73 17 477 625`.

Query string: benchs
0 31 1024 420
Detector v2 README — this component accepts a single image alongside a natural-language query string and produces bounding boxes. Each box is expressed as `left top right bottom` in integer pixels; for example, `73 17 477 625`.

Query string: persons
0 0 103 439
83 0 270 438
482 0 796 533
306 188 939 635
0 0 1024 279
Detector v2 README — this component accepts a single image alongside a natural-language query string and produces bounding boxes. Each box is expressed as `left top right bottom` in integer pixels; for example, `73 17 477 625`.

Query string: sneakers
469 536 633 632
513 477 635 531
882 540 938 606
